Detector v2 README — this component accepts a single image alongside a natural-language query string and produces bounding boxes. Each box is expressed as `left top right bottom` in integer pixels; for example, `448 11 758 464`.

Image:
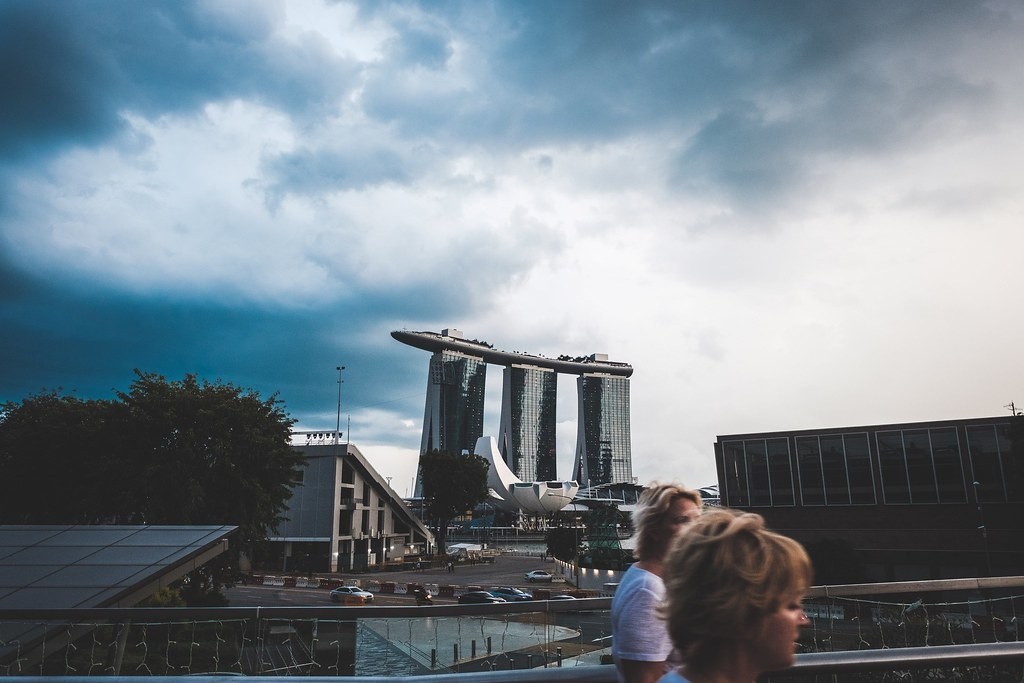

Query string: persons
441 560 454 574
540 553 546 561
657 509 813 683
412 562 424 573
610 484 701 683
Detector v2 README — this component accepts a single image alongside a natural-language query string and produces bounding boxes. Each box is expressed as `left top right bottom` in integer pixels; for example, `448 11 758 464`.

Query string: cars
550 595 576 600
329 586 374 603
490 587 532 602
523 570 552 583
457 591 506 603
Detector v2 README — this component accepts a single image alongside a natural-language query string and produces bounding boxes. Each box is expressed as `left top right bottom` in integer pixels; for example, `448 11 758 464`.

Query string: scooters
413 589 433 606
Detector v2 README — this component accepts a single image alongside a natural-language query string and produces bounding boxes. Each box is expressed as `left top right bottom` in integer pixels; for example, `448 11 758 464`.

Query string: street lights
548 492 579 594
972 481 991 578
337 365 345 432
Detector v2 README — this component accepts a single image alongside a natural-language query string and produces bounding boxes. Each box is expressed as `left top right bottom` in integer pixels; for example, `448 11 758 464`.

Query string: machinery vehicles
478 551 495 564
